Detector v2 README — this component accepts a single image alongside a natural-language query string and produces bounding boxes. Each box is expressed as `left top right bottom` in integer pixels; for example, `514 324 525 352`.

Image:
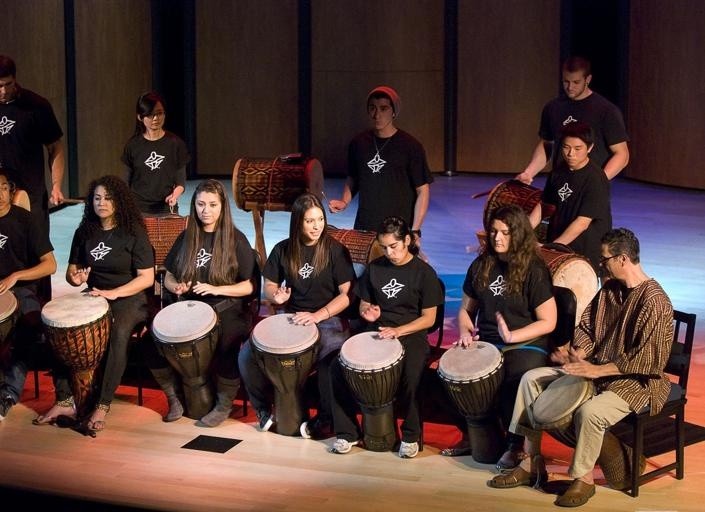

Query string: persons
528 121 614 283
1 170 57 423
119 89 191 218
0 52 68 303
330 86 434 260
138 178 261 428
442 205 560 470
487 226 677 507
35 173 157 433
231 192 358 443
321 215 444 459
516 55 633 188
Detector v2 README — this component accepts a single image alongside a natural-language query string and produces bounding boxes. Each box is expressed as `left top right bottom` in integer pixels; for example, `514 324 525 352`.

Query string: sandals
88 404 109 432
33 395 78 425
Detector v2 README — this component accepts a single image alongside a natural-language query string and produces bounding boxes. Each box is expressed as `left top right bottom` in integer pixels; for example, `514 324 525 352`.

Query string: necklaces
373 137 392 156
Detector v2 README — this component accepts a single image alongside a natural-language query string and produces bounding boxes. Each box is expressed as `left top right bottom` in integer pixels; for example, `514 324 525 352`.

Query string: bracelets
410 227 422 237
323 304 332 319
341 198 350 207
508 329 515 344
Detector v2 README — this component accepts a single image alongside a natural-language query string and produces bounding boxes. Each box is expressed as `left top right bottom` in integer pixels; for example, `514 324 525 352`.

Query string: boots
202 375 241 426
151 367 185 421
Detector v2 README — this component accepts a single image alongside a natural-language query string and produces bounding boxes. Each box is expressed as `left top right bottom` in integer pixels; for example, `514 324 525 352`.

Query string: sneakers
300 416 325 439
1 397 12 421
492 457 548 488
555 480 596 506
261 414 274 431
332 439 359 454
398 442 418 458
443 445 472 456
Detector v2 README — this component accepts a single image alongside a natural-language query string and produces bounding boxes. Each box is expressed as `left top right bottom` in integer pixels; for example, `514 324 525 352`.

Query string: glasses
146 112 167 119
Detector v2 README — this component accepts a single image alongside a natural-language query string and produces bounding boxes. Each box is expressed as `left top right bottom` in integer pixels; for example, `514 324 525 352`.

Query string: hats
366 87 401 119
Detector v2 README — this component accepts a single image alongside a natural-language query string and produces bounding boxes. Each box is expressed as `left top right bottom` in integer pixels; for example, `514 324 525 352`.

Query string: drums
532 244 600 330
336 330 406 452
139 215 190 266
322 224 386 263
532 372 648 491
0 286 19 343
39 292 114 418
249 312 320 437
8 187 32 213
481 176 553 238
230 156 325 212
438 340 506 464
150 299 224 421
